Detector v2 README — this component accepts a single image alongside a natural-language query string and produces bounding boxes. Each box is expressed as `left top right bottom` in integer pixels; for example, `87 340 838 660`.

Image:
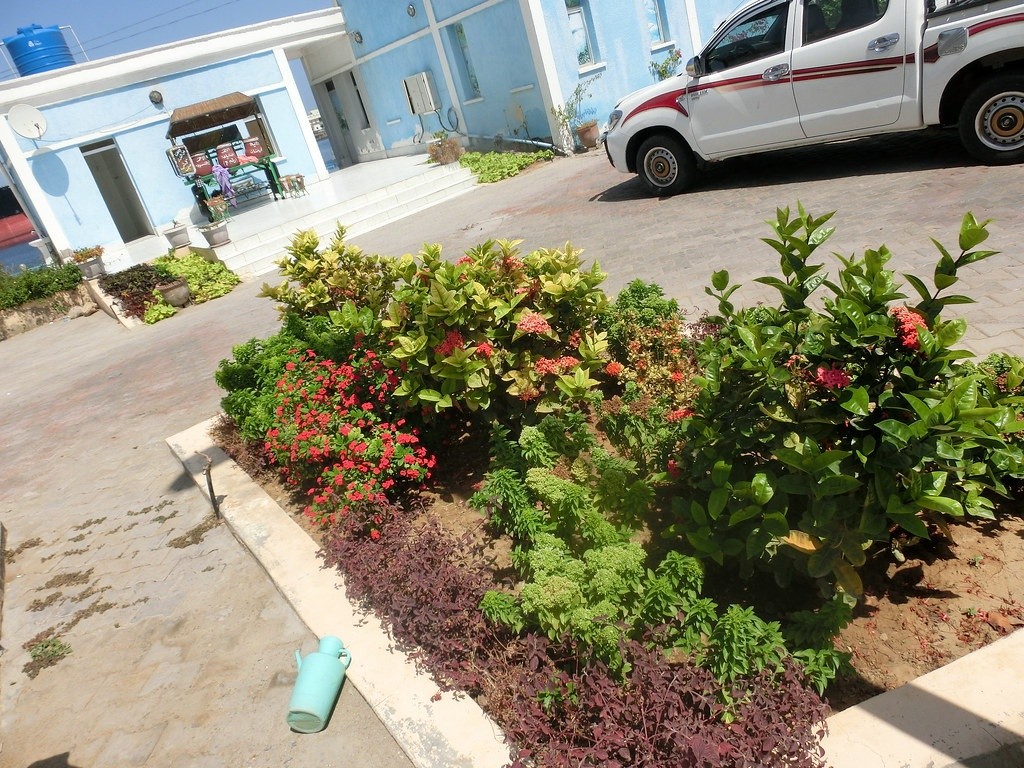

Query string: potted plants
70 245 107 282
196 219 232 250
550 73 603 149
162 219 192 251
154 265 190 306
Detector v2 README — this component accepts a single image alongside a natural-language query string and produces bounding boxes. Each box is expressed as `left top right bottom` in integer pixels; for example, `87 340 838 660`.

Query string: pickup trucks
599 0 1024 194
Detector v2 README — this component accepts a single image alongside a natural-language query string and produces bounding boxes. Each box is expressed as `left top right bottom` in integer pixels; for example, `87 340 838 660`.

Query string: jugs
287 637 352 733
